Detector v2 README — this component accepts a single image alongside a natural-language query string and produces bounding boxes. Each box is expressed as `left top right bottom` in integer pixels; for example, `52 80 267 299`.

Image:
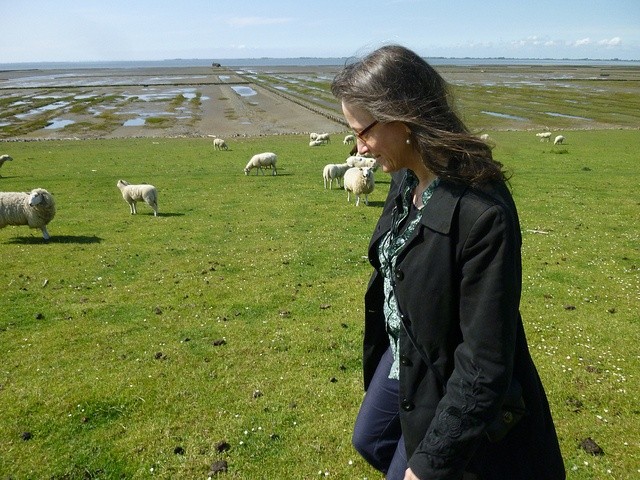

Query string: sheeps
323 161 355 190
350 143 358 156
117 179 158 217
309 140 324 146
553 135 566 145
343 167 375 206
311 133 319 141
244 152 277 175
317 133 333 144
346 155 377 171
481 134 489 140
0 154 13 177
343 135 356 145
536 132 553 143
0 188 56 239
213 138 228 151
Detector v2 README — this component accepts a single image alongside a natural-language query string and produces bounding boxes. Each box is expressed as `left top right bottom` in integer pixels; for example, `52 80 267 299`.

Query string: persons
330 40 565 480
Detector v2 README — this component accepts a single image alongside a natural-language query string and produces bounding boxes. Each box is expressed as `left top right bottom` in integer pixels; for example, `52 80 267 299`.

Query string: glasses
353 119 379 144
